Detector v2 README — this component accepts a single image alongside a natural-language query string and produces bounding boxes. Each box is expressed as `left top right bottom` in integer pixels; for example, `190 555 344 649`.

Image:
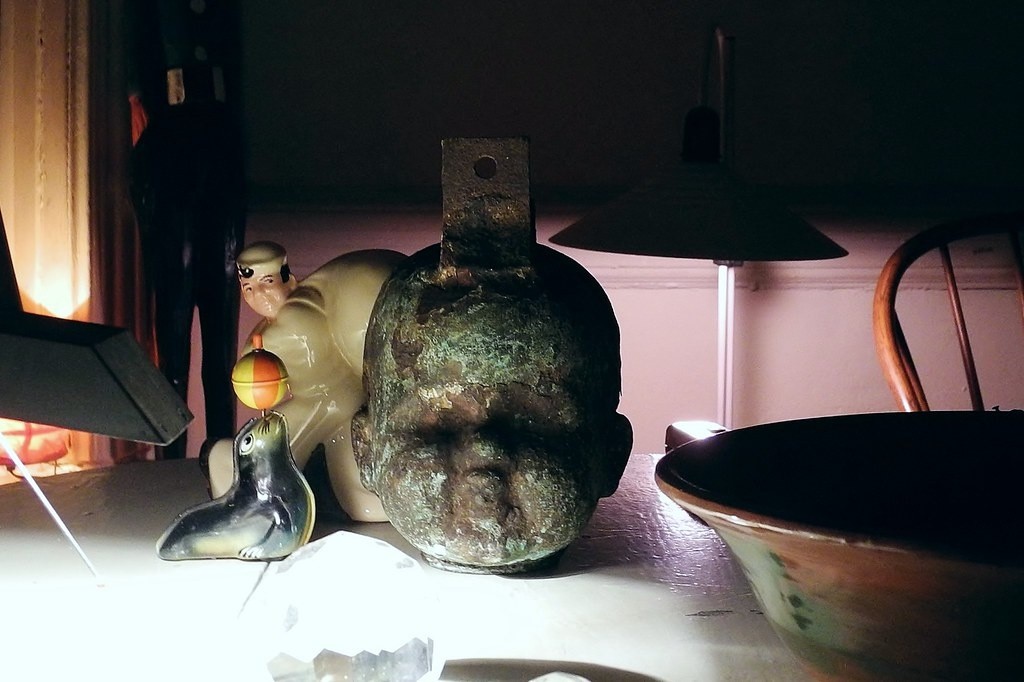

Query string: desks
0 462 814 682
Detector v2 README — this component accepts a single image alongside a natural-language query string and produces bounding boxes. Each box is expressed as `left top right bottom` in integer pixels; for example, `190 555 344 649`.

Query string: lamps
548 23 849 269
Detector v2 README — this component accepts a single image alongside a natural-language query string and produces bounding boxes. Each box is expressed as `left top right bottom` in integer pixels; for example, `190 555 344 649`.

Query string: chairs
871 212 1023 411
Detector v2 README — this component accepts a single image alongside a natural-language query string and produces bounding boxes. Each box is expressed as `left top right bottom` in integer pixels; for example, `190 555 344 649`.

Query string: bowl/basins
654 409 1024 682
